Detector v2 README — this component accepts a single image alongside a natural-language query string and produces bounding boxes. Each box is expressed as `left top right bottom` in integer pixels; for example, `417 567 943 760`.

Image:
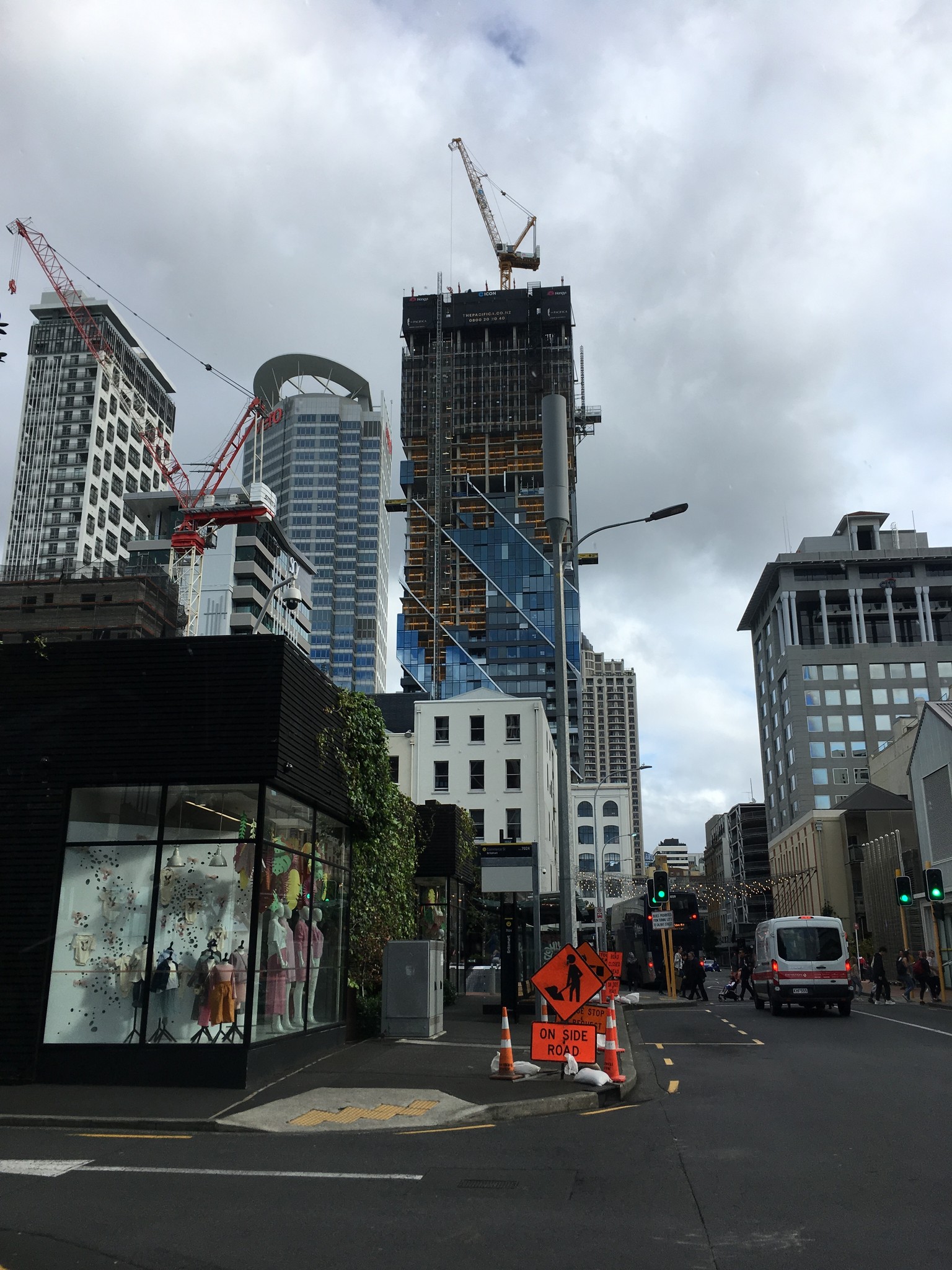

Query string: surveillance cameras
283 588 302 610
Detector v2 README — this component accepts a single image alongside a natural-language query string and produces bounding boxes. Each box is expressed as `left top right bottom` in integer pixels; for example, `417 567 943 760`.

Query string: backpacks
913 960 923 975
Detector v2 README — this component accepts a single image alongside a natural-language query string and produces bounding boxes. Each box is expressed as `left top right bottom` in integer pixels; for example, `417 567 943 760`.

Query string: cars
703 959 722 972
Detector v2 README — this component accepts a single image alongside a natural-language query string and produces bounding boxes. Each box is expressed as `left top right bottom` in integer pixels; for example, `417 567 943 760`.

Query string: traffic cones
603 1007 626 1083
489 1007 525 1081
596 982 610 1004
597 993 626 1053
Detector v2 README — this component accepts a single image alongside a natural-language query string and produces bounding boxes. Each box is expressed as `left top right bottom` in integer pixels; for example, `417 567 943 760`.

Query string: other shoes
686 997 693 1000
660 992 666 995
749 997 755 1000
741 997 744 1001
920 1001 926 1006
934 997 940 1001
721 992 725 994
719 993 722 994
701 998 708 1001
868 998 875 1003
856 997 864 1002
902 993 910 1001
678 995 686 998
695 995 702 999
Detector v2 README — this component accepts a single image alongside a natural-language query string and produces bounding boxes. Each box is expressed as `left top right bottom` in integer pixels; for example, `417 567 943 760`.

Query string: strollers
718 970 743 1002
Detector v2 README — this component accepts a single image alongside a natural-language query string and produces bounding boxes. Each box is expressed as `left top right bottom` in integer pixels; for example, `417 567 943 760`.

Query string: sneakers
886 1000 896 1005
875 1000 884 1005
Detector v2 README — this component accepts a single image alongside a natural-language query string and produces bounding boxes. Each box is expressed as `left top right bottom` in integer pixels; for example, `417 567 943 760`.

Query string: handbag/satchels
896 957 906 972
675 970 680 976
863 966 867 970
747 968 753 974
194 988 201 996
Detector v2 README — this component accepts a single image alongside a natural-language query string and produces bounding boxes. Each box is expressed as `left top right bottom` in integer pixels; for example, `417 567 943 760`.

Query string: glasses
930 953 935 954
739 952 742 954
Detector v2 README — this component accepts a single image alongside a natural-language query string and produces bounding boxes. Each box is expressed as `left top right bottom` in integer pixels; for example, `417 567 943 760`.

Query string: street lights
603 833 637 952
558 500 689 949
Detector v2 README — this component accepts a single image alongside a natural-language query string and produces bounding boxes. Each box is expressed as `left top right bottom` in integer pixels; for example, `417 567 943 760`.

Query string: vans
749 915 855 1016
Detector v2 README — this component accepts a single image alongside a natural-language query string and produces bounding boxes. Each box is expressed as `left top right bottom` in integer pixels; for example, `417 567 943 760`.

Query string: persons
731 953 739 973
868 956 886 1004
737 949 754 1001
857 955 873 983
918 951 939 1004
849 957 864 1003
491 950 501 967
926 950 941 1000
658 949 675 996
674 945 684 995
626 952 639 991
686 952 709 1001
129 903 324 1033
719 977 735 995
900 952 915 1002
873 947 896 1005
678 952 702 1000
896 949 906 990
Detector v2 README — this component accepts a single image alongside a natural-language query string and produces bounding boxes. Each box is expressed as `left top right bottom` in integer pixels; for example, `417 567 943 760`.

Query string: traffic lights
653 870 669 903
896 876 913 907
646 878 663 908
926 868 945 901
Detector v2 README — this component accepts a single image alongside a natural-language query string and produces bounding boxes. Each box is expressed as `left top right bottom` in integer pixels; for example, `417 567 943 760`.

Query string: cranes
4 216 272 636
447 136 541 290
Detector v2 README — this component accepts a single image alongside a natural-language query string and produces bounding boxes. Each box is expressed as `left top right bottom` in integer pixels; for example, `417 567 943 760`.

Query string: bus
606 891 702 990
